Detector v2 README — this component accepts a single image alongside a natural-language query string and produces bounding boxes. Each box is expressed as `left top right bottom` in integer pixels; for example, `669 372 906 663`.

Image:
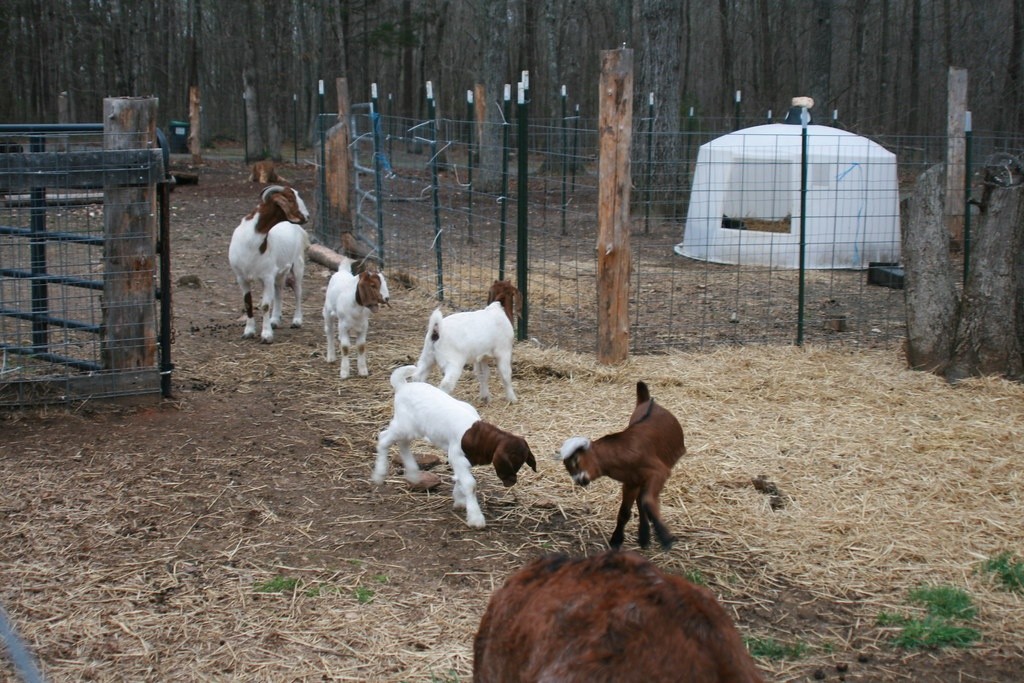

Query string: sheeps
228 185 312 346
472 551 770 682
411 279 522 406
372 365 537 531
558 379 687 551
322 256 390 381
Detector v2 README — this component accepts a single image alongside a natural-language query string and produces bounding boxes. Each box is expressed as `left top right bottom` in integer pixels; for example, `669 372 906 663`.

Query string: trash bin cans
169 119 191 154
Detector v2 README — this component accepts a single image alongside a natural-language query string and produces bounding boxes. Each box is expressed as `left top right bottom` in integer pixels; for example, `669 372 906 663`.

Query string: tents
674 97 905 270
